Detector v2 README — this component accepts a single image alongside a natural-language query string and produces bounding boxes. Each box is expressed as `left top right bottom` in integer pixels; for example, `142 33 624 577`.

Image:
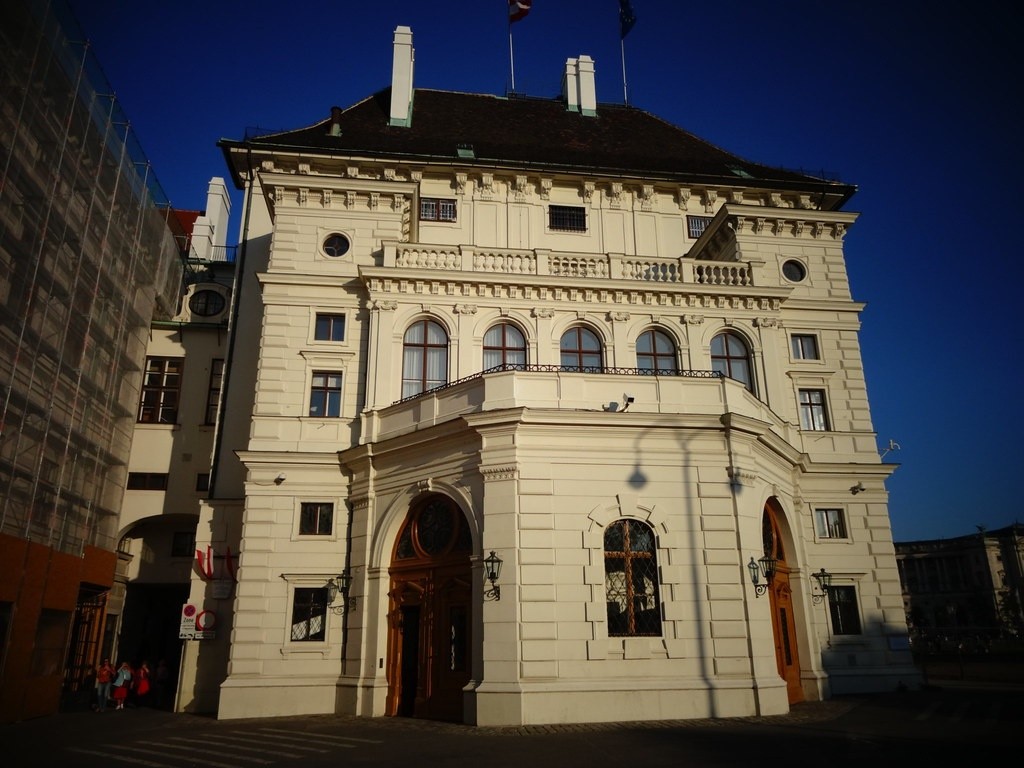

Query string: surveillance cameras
278 474 286 481
858 480 865 491
623 392 635 403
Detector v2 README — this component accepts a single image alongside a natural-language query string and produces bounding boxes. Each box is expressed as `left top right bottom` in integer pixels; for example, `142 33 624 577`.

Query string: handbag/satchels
123 679 130 688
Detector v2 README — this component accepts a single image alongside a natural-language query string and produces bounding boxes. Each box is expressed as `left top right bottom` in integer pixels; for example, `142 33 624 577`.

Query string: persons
92 657 171 713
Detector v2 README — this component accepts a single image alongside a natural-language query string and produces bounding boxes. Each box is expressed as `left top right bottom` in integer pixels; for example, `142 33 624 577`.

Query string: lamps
278 472 287 481
337 569 358 610
748 557 765 598
485 550 502 600
452 137 479 158
810 568 832 603
759 552 774 589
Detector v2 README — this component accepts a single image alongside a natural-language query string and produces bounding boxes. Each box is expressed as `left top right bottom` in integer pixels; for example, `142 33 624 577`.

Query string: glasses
124 666 127 667
104 661 108 662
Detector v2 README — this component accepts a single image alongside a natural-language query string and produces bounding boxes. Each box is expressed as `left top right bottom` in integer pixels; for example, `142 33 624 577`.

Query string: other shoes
121 703 124 709
95 708 100 712
115 705 120 710
101 709 104 712
